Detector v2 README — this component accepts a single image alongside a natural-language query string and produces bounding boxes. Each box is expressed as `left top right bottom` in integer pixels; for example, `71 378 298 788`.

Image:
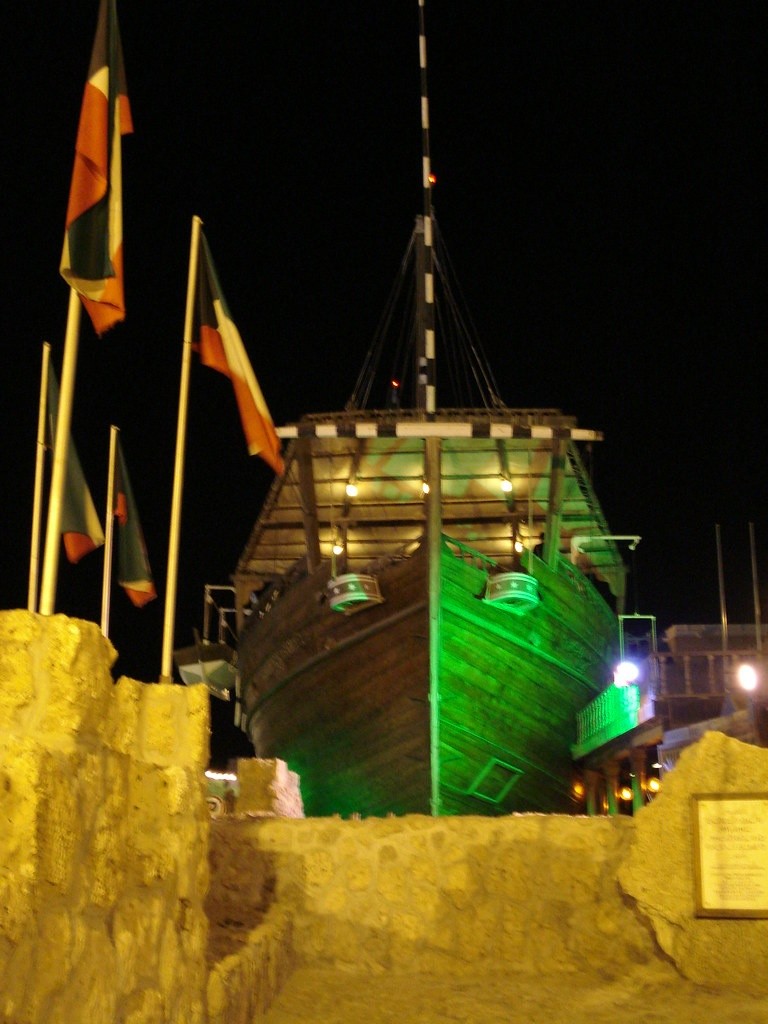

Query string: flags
41 359 154 604
64 0 137 333
193 233 288 478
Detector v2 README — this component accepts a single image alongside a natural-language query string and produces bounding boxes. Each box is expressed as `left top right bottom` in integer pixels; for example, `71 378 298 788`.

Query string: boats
171 631 238 704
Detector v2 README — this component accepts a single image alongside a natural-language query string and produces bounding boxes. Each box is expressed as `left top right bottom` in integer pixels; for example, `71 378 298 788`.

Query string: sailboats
647 519 768 773
169 0 662 816
